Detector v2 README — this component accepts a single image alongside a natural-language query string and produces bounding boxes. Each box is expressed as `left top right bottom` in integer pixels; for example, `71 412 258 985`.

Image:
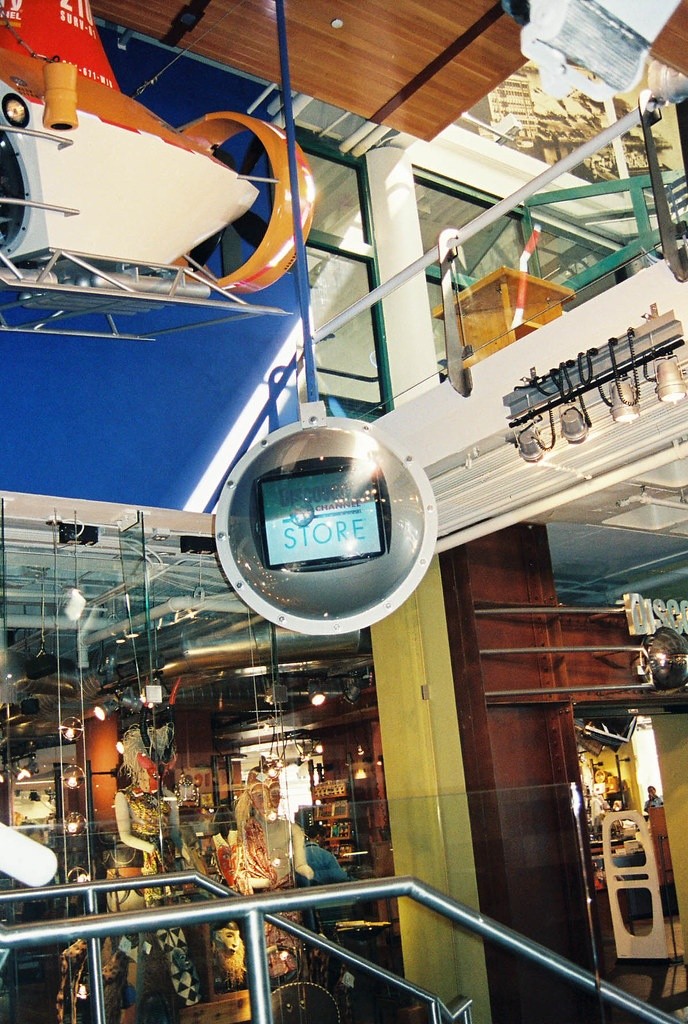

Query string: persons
644 786 663 840
297 826 347 999
103 766 314 1023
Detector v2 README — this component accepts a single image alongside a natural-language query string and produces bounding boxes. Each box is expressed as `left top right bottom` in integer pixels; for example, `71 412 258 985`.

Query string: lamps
20 699 40 716
308 678 326 706
376 755 383 766
344 678 365 706
352 726 364 755
119 687 143 713
515 429 547 462
608 377 641 423
24 652 59 679
653 353 688 402
295 758 305 766
21 762 38 780
560 401 588 444
95 699 119 722
0 770 9 783
268 729 287 776
265 683 288 705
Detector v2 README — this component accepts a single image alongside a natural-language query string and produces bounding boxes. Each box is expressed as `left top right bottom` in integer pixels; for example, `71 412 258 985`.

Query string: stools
328 926 392 1024
